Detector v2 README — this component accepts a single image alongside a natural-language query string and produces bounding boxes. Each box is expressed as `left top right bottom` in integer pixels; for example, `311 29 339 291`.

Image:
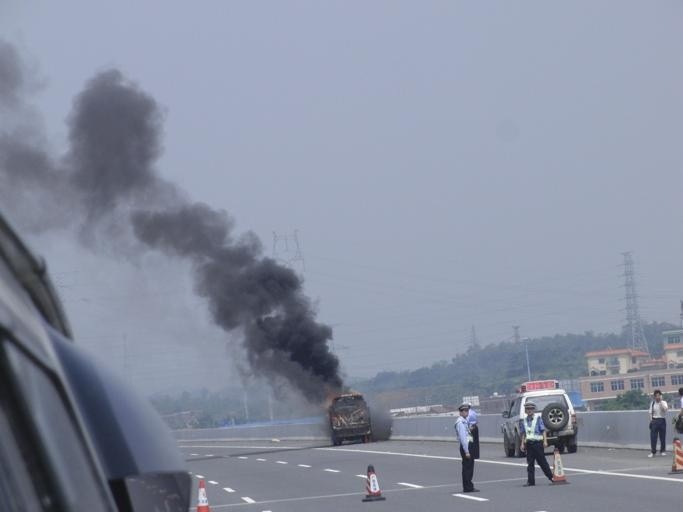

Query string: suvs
499 378 580 458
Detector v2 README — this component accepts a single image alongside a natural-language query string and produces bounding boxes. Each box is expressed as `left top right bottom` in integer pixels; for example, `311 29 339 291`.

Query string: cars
0 209 198 511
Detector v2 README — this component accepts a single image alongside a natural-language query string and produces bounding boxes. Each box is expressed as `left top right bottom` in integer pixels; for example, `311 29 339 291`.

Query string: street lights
521 337 532 381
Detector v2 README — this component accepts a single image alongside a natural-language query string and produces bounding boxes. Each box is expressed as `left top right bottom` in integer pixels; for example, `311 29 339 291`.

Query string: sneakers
660 452 667 456
648 452 656 458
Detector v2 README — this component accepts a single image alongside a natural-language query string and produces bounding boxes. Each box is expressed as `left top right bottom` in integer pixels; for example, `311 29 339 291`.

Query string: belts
527 440 542 444
652 417 665 420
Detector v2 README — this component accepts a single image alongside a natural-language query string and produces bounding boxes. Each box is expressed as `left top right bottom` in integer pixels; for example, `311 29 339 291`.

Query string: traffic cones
196 478 209 511
550 447 571 487
362 464 388 504
666 437 682 475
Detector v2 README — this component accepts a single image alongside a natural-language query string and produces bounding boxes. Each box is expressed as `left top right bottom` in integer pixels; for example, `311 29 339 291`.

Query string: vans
328 393 374 447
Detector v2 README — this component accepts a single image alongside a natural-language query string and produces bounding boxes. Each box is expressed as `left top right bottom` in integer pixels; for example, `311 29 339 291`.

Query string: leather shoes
522 481 535 487
463 488 480 492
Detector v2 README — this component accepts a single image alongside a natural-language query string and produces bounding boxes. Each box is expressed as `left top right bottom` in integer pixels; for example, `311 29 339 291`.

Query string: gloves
465 452 471 459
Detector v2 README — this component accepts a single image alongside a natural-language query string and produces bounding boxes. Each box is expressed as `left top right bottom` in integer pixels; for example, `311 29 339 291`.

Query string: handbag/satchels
676 416 683 434
648 421 652 430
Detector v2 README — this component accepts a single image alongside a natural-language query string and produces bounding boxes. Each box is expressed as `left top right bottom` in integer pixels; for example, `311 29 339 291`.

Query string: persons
466 403 481 460
645 389 668 458
452 401 480 493
672 386 683 425
517 400 553 488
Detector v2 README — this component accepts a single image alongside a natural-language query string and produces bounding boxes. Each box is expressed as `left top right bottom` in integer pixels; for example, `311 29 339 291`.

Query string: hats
523 400 536 408
457 401 471 411
